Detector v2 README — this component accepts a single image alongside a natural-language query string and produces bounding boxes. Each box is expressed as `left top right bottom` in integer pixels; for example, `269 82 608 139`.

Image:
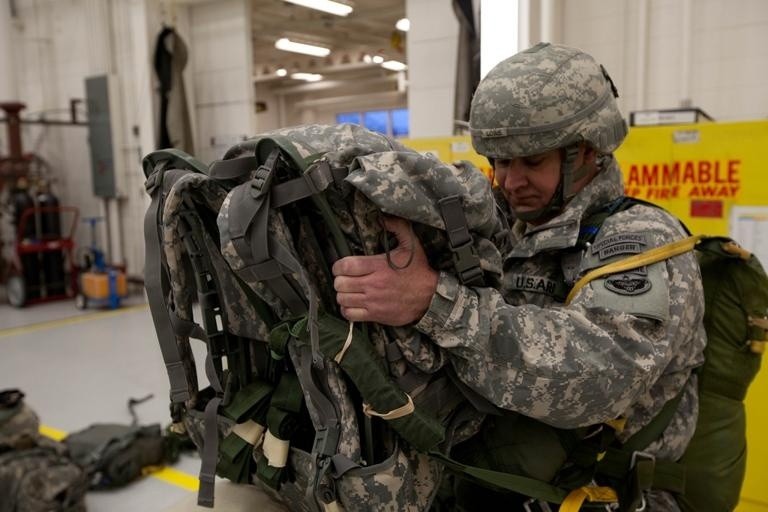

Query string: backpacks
1 443 88 512
141 123 500 511
564 194 766 510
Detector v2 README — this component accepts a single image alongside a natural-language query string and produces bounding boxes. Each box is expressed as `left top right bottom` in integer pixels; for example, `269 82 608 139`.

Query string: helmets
466 38 630 161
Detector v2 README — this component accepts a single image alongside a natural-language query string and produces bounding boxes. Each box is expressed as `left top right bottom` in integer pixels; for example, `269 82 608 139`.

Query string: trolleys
6 206 79 307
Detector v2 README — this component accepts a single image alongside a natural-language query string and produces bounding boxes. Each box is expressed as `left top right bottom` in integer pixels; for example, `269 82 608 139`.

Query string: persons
332 43 706 510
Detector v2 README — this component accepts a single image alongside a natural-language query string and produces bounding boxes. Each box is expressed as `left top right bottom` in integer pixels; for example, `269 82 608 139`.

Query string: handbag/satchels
57 422 164 490
